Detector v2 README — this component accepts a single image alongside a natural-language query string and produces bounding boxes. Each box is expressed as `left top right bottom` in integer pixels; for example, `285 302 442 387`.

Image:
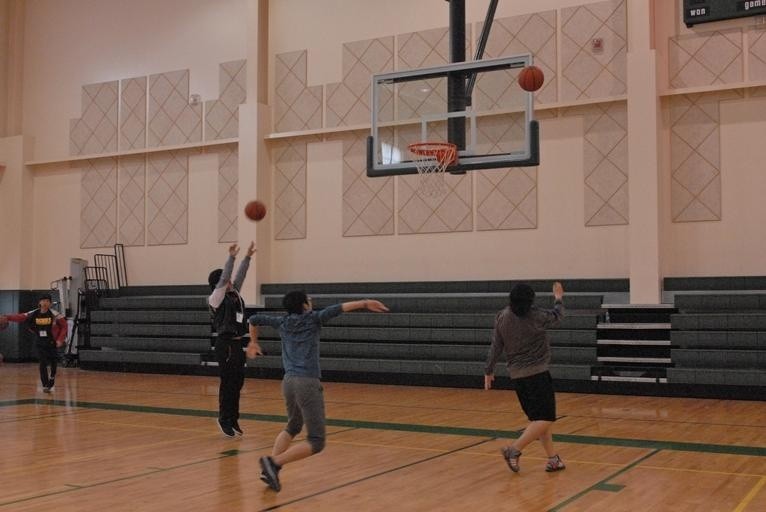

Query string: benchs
79 295 213 368
666 288 766 387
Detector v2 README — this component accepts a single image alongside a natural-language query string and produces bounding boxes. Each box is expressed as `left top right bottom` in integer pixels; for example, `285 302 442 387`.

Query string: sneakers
502 446 521 472
218 417 242 437
546 455 565 472
260 456 282 491
43 378 55 393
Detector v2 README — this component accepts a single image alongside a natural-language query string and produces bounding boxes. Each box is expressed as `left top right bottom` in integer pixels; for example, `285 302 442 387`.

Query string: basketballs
245 201 265 221
520 67 544 92
0 317 9 329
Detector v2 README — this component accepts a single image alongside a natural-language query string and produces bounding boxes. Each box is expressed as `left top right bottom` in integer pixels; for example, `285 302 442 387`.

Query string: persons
247 280 389 492
482 280 566 472
3 293 68 394
206 241 258 440
